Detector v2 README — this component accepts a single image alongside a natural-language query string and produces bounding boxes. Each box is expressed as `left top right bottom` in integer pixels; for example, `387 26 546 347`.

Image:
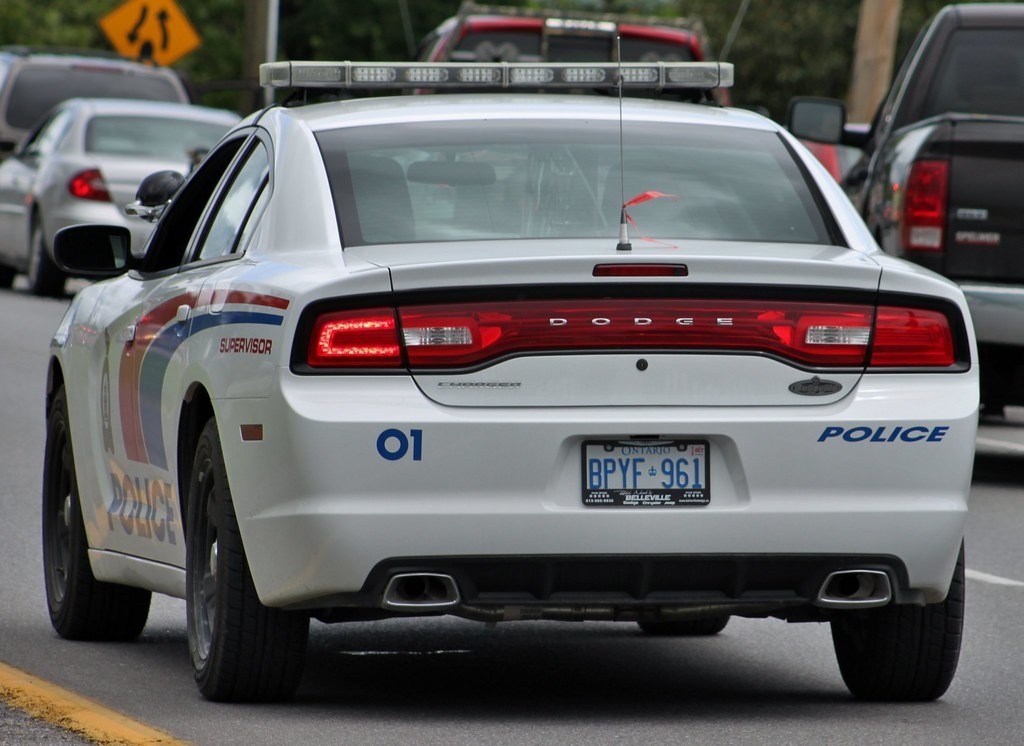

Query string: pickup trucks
835 1 1023 404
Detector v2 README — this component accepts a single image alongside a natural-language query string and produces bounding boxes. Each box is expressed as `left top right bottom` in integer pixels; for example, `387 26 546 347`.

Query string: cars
0 101 249 292
0 43 187 159
40 58 982 702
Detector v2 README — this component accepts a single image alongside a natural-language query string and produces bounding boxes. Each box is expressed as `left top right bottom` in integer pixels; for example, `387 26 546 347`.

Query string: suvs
402 0 733 106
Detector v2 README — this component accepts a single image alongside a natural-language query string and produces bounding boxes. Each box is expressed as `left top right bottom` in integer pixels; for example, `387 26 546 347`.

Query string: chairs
347 154 417 246
602 162 702 232
